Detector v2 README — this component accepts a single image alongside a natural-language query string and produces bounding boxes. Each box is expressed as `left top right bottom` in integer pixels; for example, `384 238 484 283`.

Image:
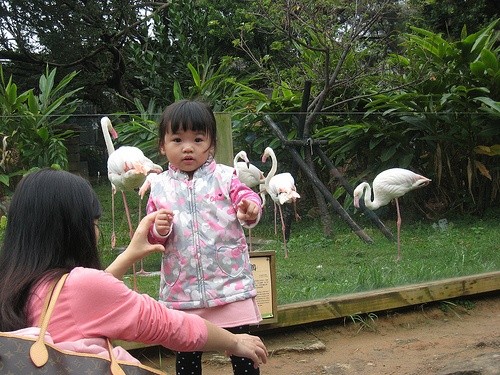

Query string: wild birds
265 147 303 260
354 168 432 262
101 117 164 292
234 150 265 252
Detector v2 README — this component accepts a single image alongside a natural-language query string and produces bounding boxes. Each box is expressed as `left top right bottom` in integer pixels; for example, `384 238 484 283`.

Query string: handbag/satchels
0 271 166 375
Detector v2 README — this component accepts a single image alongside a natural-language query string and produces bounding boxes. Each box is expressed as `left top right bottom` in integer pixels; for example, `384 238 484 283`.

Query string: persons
145 100 262 375
0 167 268 375
